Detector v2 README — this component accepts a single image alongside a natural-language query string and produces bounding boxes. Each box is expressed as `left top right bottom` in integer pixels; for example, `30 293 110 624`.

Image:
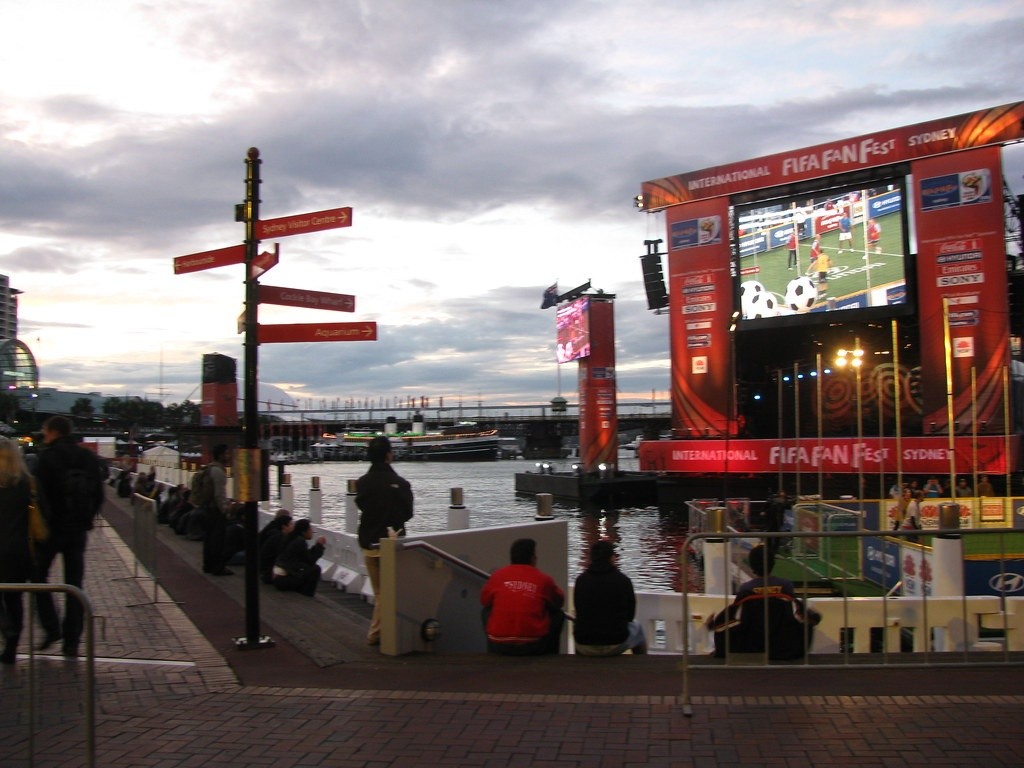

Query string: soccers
741 281 777 319
786 276 816 310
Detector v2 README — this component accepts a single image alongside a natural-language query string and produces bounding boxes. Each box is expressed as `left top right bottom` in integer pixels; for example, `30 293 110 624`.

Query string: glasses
612 553 620 557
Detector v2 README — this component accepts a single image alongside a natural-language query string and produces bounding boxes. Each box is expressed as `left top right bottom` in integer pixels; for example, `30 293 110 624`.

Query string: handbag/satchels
27 476 49 539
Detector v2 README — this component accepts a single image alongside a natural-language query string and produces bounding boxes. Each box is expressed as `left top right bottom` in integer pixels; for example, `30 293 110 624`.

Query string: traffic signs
251 241 280 278
172 243 244 275
257 321 377 343
257 285 355 312
255 206 353 240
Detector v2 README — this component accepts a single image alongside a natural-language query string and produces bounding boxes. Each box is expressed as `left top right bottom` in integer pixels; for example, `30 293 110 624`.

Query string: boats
563 435 643 460
310 428 526 459
269 449 297 462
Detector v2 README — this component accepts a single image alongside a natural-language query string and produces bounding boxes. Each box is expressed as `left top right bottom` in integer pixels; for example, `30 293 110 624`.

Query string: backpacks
191 463 226 506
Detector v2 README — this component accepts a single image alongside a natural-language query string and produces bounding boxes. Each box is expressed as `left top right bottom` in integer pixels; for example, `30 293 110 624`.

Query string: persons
571 540 651 656
111 444 327 599
735 186 882 297
0 436 36 666
352 437 414 644
706 542 824 657
890 474 995 530
29 416 107 664
478 539 565 656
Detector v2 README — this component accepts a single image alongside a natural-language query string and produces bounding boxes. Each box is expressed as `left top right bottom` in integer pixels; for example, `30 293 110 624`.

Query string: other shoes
0 649 15 664
61 628 79 638
37 630 60 652
